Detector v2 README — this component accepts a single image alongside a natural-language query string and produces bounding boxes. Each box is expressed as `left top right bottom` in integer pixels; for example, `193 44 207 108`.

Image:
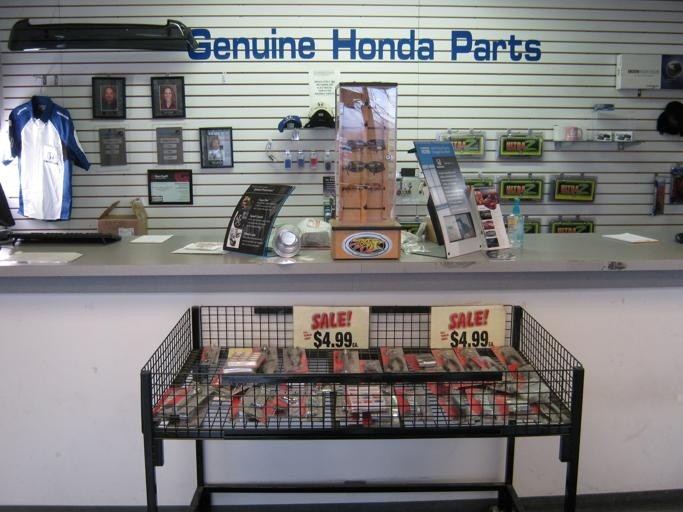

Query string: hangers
32 76 48 112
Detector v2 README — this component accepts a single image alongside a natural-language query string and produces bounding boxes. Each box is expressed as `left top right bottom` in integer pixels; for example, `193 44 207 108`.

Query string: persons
160 86 176 110
207 137 223 160
101 87 116 110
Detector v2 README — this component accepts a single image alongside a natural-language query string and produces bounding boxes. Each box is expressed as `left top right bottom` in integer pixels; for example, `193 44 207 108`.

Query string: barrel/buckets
271 224 303 258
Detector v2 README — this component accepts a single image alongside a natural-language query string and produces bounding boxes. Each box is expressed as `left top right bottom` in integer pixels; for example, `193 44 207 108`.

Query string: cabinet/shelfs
140 304 584 512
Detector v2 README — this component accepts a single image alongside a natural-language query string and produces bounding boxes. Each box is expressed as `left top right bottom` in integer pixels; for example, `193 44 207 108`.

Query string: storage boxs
97 200 147 237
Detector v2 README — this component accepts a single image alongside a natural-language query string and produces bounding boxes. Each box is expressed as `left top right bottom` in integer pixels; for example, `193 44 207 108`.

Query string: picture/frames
151 76 186 118
199 127 233 168
147 169 193 205
92 76 126 119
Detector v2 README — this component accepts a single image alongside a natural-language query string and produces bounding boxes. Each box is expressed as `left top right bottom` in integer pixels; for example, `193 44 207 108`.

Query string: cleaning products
505 197 525 250
